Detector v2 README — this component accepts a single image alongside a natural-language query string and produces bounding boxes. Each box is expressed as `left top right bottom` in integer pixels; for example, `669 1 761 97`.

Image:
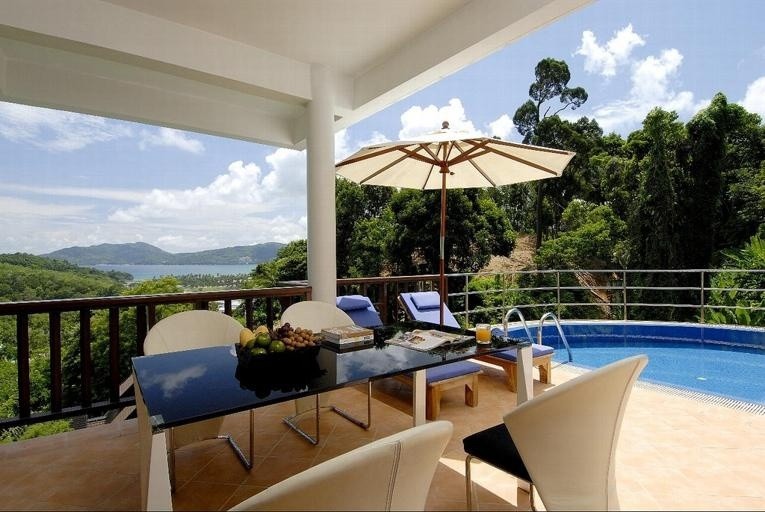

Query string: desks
133 320 533 493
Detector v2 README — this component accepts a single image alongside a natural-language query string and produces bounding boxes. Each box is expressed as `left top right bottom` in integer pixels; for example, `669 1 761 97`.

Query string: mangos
239 326 270 346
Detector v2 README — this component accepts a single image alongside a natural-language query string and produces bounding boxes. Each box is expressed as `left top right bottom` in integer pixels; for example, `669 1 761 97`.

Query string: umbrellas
334 118 578 322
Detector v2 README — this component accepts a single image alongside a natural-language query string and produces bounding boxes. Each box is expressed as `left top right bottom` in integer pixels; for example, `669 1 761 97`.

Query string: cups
475 322 493 345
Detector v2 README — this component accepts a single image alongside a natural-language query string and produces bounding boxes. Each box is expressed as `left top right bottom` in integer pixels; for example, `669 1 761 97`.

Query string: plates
235 341 321 367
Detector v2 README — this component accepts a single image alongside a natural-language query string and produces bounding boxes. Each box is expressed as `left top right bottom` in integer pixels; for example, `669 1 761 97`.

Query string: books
320 323 374 339
321 333 373 345
383 328 477 353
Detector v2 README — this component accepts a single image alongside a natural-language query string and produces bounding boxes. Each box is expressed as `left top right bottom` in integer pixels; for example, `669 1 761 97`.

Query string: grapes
270 322 322 352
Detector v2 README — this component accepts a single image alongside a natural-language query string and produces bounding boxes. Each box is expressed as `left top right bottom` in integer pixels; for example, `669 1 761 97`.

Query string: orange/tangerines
246 332 285 357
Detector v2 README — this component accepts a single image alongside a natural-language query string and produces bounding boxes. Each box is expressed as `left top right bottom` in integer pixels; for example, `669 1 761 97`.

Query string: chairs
396 291 556 392
461 352 649 510
129 309 258 480
142 421 455 511
337 293 484 422
279 299 373 445
